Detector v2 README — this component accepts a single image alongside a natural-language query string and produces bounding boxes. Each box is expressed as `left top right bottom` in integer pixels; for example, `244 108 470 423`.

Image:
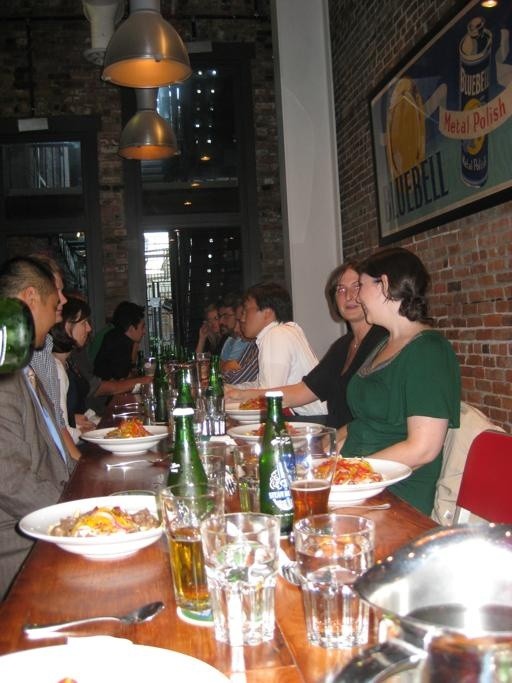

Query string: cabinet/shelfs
0 115 106 340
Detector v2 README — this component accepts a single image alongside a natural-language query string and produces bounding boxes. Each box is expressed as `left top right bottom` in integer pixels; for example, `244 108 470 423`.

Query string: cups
192 437 266 514
201 511 280 645
292 515 372 647
286 426 337 531
161 484 223 616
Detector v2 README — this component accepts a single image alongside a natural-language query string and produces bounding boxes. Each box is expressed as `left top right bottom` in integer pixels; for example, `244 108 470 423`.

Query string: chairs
453 429 512 537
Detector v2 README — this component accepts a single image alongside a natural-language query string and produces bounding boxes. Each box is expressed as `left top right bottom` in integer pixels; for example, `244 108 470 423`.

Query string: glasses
215 313 233 320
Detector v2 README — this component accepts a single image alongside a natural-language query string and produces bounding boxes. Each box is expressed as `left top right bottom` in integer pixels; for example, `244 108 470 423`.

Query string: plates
1 644 232 683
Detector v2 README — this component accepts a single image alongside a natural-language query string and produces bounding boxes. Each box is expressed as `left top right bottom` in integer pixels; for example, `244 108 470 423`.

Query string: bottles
0 297 37 373
137 341 224 427
259 390 294 536
165 408 210 516
137 341 296 539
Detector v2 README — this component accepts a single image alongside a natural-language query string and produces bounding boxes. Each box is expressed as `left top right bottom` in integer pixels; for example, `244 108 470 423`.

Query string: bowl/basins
225 401 290 426
79 426 166 456
18 495 162 560
309 456 412 509
228 422 323 455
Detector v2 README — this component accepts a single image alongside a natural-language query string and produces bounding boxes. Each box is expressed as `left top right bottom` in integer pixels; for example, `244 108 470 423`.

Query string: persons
26 254 82 462
194 282 329 427
67 344 154 415
95 300 146 406
1 254 78 601
49 297 112 425
223 258 390 453
82 302 138 373
52 353 97 445
314 247 462 566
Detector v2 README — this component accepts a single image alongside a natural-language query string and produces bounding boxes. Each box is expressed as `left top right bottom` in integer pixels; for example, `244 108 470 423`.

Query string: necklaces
351 338 362 353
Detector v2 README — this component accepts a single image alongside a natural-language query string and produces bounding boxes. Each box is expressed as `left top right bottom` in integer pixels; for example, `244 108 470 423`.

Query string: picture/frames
367 0 511 246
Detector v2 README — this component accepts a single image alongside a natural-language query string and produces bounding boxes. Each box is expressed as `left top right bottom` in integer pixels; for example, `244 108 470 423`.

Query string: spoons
24 602 165 634
105 455 172 468
113 396 153 408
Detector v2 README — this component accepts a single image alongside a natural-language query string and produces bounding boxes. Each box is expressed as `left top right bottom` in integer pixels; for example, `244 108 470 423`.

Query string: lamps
117 87 178 160
102 1 190 90
